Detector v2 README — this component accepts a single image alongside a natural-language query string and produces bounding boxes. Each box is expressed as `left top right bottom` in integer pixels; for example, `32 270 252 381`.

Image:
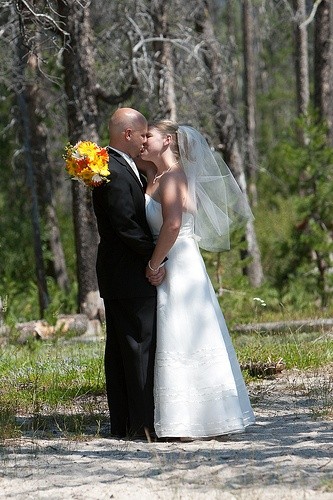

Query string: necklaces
151 161 178 184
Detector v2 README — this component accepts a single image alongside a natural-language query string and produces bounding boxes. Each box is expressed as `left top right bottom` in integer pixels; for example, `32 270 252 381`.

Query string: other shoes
135 430 170 443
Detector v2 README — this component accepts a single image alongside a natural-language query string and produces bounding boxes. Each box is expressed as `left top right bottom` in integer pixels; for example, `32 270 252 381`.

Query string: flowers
58 138 111 191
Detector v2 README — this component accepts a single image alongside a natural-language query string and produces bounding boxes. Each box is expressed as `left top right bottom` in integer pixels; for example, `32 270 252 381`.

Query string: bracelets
147 256 168 272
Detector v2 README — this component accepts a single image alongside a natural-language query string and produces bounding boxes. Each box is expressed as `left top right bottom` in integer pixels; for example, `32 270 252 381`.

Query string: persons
88 106 168 441
138 118 257 440
